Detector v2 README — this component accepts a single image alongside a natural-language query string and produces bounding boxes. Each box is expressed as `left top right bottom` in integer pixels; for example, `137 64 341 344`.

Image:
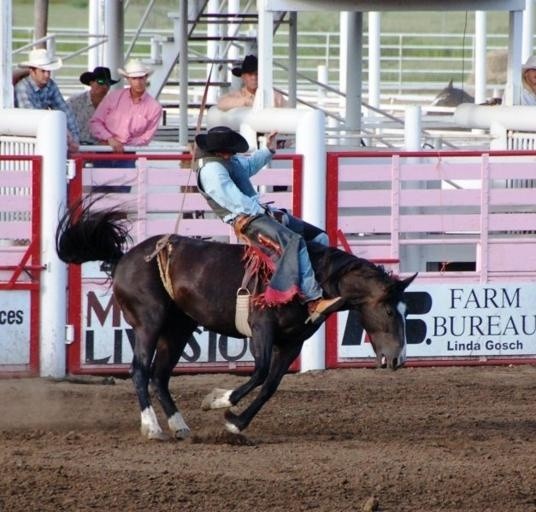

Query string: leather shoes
308 297 344 323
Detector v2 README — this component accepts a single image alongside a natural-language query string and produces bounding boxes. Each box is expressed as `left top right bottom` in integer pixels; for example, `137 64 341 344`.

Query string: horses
55 175 419 441
426 78 502 115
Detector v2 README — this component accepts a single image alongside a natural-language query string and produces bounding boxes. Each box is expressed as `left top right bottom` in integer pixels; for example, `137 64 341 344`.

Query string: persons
14 48 80 155
496 52 536 105
215 54 292 192
191 126 345 324
63 65 121 145
89 58 162 221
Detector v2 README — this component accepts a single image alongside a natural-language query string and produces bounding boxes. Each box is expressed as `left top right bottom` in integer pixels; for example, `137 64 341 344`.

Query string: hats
80 66 120 87
118 60 155 77
196 126 249 153
18 49 62 71
522 55 536 71
232 55 259 77
